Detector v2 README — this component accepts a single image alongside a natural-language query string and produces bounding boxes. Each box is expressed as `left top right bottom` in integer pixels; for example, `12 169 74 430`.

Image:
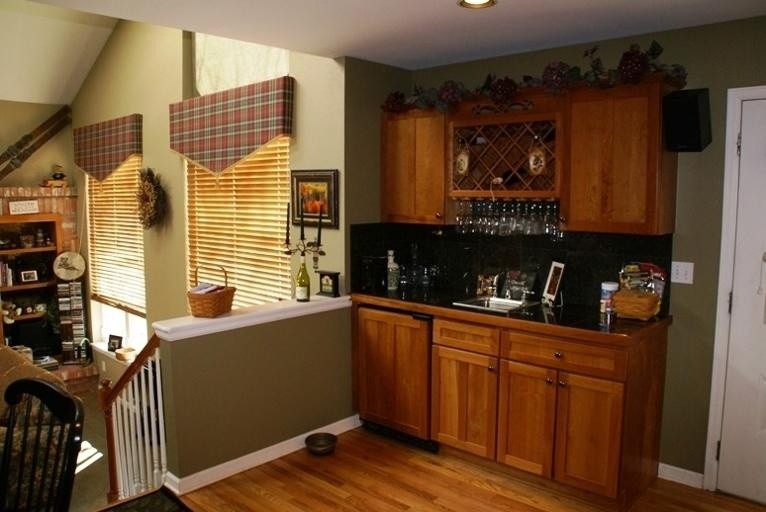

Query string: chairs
0 378 85 512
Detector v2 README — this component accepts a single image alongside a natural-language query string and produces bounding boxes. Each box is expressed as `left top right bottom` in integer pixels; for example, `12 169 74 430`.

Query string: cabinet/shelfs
381 101 448 227
431 319 501 463
497 335 632 500
560 76 676 235
447 86 560 198
355 308 429 441
0 213 78 363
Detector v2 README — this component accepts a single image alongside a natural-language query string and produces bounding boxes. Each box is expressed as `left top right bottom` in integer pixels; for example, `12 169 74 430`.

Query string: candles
317 205 322 245
300 199 304 240
286 202 290 243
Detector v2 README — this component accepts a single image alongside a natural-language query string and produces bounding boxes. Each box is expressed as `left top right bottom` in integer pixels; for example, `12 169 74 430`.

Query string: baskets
613 287 661 322
185 262 238 319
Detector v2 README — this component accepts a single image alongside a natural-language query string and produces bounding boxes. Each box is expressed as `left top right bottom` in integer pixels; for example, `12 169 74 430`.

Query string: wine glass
455 201 558 236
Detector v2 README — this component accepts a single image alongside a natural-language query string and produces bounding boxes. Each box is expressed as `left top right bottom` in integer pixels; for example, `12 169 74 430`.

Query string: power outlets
670 262 694 284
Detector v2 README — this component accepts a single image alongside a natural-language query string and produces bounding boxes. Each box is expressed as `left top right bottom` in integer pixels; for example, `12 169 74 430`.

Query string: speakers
660 87 713 153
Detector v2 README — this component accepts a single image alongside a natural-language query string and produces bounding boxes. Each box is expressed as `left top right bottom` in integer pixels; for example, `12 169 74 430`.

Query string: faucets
492 274 499 297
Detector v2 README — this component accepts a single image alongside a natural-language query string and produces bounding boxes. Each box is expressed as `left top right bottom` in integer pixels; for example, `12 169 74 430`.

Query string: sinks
453 296 529 312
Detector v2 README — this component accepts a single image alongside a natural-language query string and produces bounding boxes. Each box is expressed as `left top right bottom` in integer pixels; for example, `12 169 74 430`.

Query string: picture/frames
21 271 38 282
291 169 339 230
108 335 122 352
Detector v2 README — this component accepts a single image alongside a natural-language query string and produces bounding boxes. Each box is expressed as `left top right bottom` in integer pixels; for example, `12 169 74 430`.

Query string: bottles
387 250 399 290
600 282 619 322
422 267 430 284
407 254 423 285
297 252 310 302
